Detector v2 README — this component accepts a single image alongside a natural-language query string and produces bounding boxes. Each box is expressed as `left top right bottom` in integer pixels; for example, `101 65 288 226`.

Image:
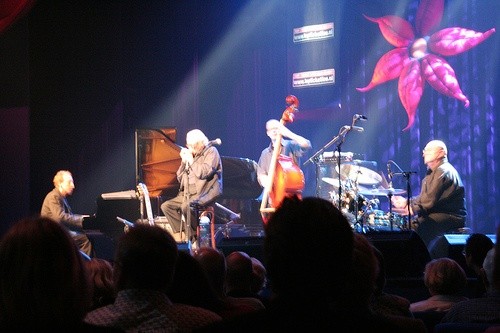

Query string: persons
391 140 467 256
84 224 222 333
0 217 92 333
409 258 472 314
41 170 96 258
256 119 312 210
194 197 426 333
192 247 265 323
435 227 500 333
338 232 412 316
85 259 115 309
166 250 208 309
161 129 223 243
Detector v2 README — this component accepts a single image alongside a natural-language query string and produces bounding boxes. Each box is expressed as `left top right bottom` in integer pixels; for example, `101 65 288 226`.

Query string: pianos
82 128 263 262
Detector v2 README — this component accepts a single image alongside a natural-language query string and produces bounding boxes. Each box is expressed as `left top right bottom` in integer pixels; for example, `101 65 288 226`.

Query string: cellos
258 94 304 224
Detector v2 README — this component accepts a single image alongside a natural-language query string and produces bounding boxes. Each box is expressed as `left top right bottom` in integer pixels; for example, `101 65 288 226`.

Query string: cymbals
322 177 367 196
335 164 382 185
360 187 407 196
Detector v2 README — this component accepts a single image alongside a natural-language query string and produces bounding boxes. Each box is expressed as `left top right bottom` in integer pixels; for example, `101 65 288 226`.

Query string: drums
364 209 403 228
336 191 372 226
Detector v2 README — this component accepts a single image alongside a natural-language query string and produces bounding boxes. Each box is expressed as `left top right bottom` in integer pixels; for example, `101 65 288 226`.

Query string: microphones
205 137 222 146
355 114 369 122
344 125 364 133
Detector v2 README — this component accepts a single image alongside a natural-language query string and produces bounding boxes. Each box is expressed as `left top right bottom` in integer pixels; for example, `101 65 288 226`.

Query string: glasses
423 149 442 153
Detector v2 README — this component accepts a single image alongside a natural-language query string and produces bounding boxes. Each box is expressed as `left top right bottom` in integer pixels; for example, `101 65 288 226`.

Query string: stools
190 206 217 250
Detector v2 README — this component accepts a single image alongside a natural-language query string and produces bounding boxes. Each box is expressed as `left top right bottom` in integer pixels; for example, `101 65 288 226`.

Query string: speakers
429 234 497 276
364 230 431 277
208 223 268 263
